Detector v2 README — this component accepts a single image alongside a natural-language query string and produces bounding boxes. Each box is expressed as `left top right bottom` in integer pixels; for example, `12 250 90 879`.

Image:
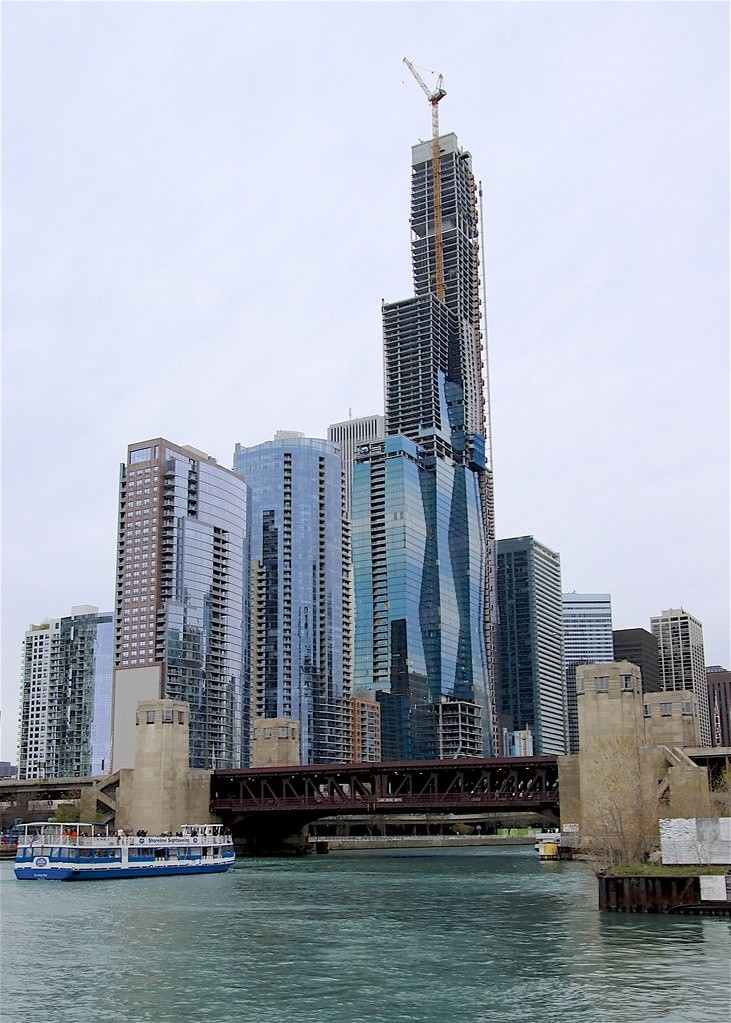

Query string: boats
0 829 41 859
13 821 235 881
534 828 561 852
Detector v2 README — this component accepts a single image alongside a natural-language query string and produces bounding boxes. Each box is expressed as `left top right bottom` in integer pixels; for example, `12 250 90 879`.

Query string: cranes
402 56 447 304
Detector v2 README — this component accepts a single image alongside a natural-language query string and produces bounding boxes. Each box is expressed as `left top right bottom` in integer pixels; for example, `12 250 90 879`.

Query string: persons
137 830 183 837
115 827 126 837
63 828 105 839
191 827 230 837
